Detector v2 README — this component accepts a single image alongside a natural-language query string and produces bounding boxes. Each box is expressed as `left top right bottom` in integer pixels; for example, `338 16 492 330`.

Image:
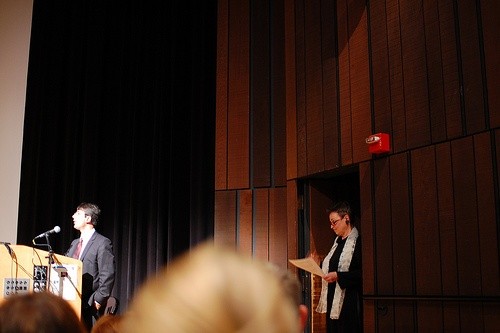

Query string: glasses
331 219 342 225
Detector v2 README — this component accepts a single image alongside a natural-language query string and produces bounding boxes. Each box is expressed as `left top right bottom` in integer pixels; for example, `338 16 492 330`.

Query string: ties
73 238 84 259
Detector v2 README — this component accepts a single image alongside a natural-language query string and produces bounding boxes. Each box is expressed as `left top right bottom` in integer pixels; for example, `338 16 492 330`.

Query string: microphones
34 226 61 240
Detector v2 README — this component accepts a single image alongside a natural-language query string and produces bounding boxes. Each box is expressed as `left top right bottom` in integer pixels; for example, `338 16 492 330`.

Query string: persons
64 203 116 333
0 290 89 333
314 206 364 333
91 314 122 333
122 238 309 333
